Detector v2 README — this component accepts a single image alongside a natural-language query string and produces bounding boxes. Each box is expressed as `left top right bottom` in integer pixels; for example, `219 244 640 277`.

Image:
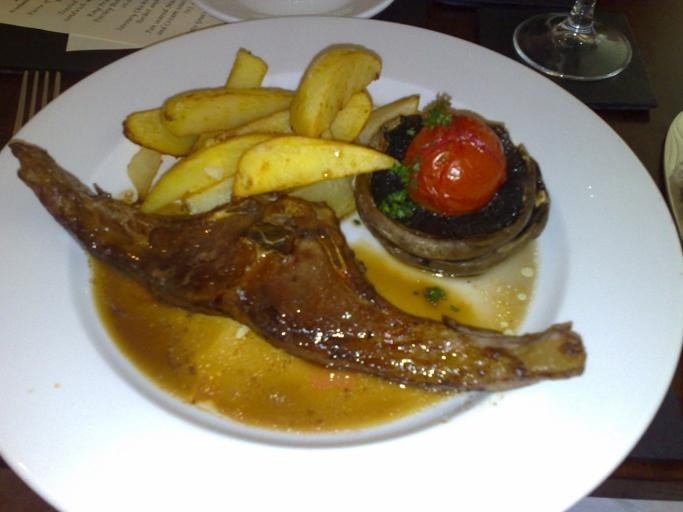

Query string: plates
195 1 394 21
1 18 681 508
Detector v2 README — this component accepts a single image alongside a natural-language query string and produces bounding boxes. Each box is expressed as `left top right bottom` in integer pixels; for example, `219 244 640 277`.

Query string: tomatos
401 108 507 218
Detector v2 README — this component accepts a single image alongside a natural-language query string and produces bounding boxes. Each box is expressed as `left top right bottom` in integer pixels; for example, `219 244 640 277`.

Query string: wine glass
511 0 636 85
